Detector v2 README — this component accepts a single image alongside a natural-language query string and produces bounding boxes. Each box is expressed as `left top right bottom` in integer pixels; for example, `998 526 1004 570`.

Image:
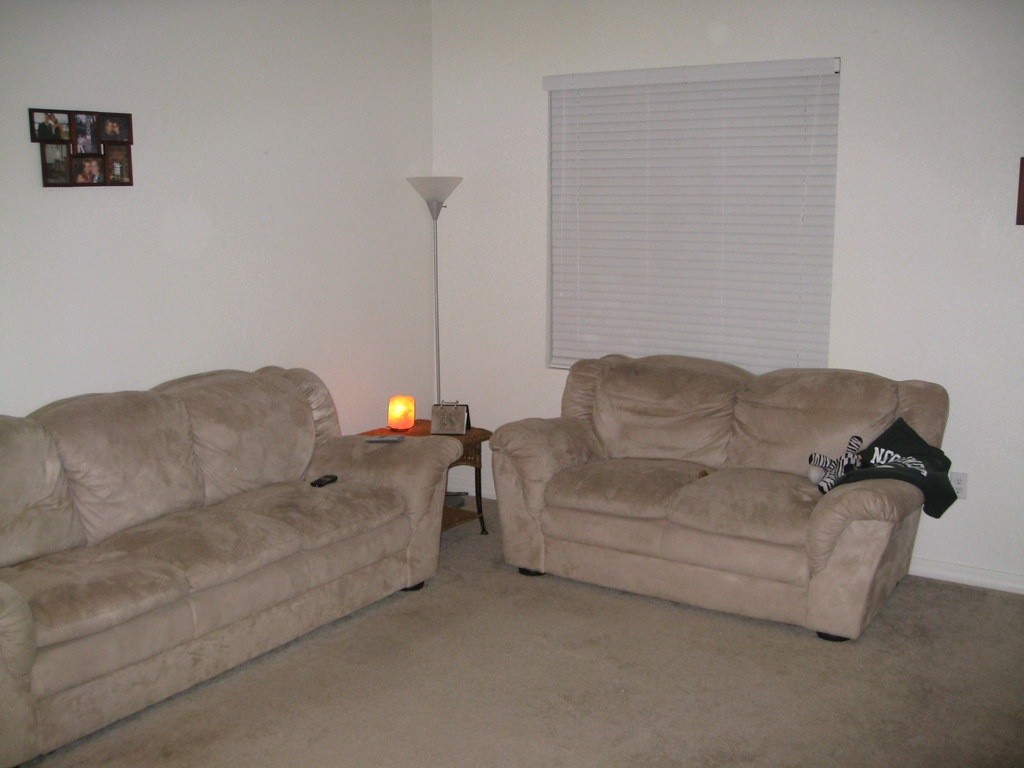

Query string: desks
358 418 493 536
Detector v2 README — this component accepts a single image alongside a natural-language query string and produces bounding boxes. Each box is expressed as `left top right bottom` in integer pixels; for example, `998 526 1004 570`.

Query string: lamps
387 394 416 433
406 176 470 514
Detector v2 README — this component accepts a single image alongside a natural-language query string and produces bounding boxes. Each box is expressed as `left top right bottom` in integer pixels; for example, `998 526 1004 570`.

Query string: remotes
312 475 338 487
365 434 403 442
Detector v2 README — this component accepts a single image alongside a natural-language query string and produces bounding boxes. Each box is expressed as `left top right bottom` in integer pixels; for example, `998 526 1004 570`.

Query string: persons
34 112 64 138
73 120 126 184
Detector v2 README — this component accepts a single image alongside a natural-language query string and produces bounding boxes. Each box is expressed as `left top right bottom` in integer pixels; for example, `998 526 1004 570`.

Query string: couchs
0 365 465 768
489 353 951 641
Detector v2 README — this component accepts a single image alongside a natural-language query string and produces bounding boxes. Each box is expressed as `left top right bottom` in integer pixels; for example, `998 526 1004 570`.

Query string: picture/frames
28 107 134 188
